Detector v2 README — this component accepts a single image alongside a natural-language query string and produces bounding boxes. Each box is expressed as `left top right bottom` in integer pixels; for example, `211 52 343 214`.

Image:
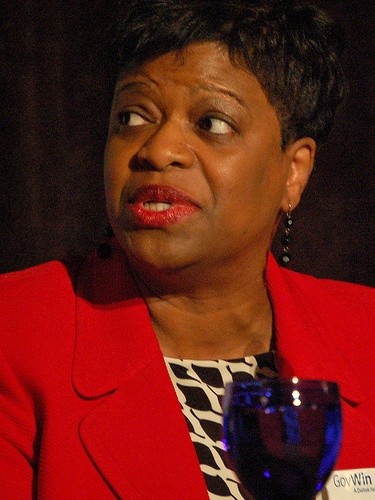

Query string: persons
1 0 375 500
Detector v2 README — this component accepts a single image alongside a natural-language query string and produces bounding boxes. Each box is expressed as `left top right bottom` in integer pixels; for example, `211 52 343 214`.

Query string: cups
223 380 343 499
